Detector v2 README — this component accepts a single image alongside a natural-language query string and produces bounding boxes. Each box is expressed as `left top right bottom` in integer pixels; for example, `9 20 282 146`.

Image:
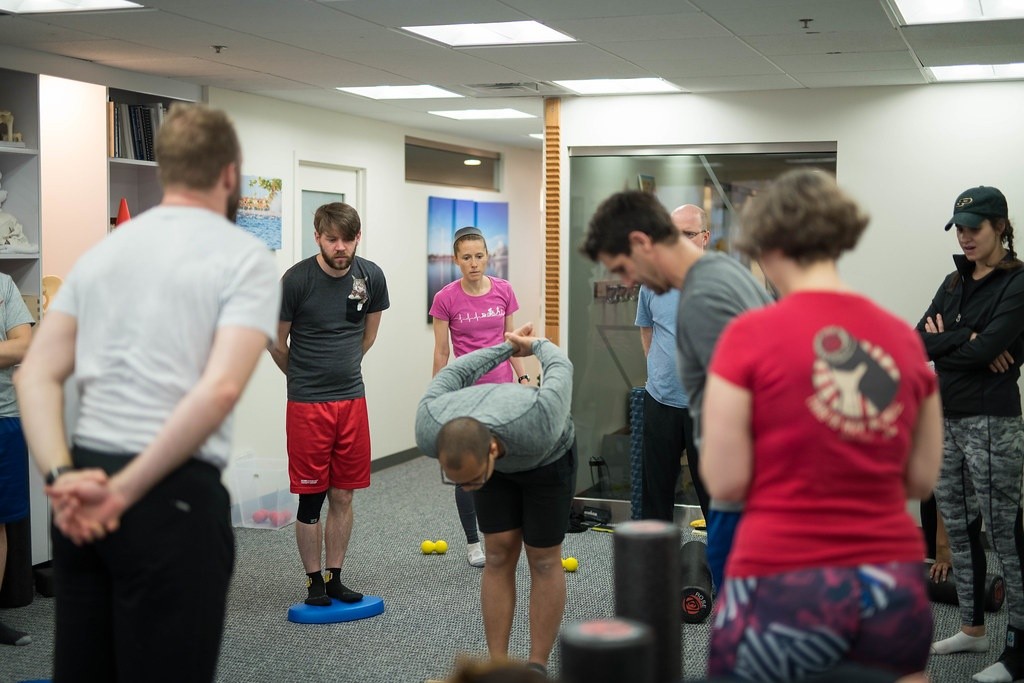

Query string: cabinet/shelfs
105 84 202 237
0 66 44 329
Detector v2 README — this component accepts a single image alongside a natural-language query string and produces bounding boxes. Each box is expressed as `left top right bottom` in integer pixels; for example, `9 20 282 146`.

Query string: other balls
562 557 579 572
420 540 448 554
252 509 291 525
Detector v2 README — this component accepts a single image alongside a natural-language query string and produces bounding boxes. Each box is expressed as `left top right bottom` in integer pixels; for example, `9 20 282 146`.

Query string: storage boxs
224 457 299 530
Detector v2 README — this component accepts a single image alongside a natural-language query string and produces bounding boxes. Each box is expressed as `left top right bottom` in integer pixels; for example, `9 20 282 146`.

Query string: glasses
682 229 707 239
440 442 491 486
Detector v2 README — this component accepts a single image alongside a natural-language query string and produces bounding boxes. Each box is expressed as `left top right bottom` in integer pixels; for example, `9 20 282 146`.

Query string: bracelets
44 464 76 485
518 373 530 383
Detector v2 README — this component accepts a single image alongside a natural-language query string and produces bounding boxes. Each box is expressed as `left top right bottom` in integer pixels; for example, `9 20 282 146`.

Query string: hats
944 186 1008 231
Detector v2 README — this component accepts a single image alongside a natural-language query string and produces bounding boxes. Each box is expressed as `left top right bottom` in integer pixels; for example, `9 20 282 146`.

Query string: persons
13 103 283 680
920 492 953 585
578 190 776 598
635 203 712 530
0 272 36 644
696 166 943 683
415 322 580 679
429 227 530 565
913 185 1024 683
266 202 390 606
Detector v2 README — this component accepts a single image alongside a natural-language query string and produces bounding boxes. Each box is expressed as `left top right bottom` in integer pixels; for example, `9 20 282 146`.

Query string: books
109 100 167 161
